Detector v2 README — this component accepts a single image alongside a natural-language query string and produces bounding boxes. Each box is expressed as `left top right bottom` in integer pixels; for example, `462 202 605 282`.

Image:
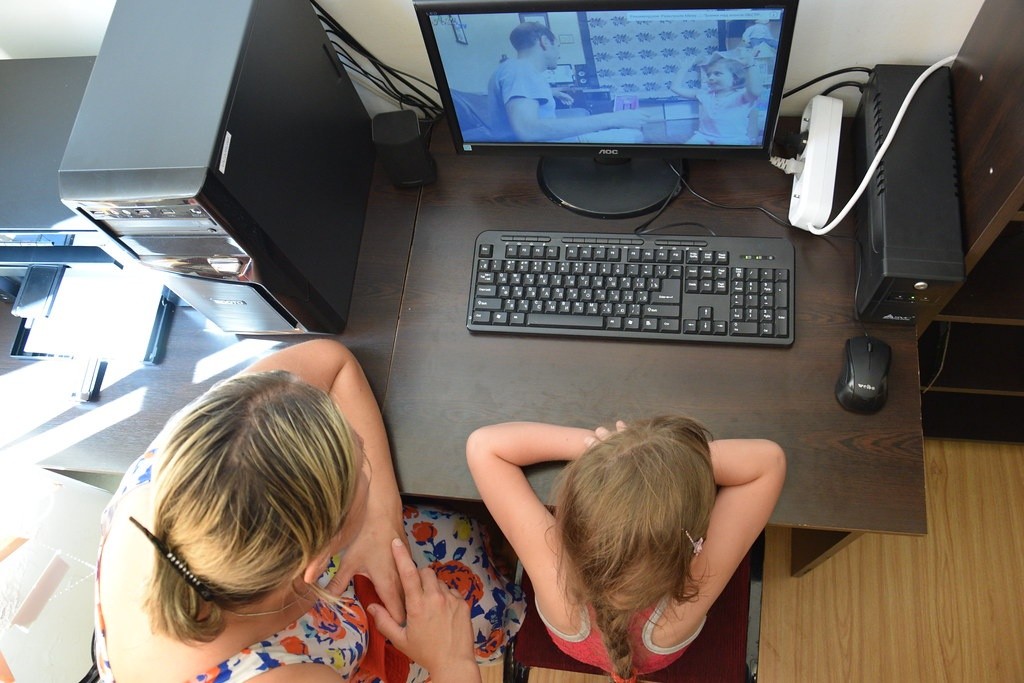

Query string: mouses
836 337 892 414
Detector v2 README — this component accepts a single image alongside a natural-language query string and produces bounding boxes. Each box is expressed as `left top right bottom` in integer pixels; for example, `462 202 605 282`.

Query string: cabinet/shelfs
917 0 1024 448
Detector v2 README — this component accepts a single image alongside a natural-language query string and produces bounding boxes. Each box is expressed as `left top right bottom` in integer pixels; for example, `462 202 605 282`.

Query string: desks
380 119 928 578
0 54 428 494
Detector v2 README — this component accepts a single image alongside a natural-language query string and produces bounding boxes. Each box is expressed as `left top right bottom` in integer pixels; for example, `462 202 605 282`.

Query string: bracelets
743 62 756 69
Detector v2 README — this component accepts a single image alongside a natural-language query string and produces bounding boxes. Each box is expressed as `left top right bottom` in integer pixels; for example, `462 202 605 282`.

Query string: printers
0 56 176 402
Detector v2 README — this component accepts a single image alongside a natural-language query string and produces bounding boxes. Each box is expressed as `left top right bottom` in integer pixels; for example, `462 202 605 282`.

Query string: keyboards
466 231 796 347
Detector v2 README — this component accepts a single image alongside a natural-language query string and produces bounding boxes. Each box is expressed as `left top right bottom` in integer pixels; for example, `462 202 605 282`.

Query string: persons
466 416 786 683
93 337 482 683
490 21 647 143
669 49 759 145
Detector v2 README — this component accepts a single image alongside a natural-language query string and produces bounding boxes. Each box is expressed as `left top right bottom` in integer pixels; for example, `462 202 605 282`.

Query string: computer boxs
848 64 964 327
58 0 373 335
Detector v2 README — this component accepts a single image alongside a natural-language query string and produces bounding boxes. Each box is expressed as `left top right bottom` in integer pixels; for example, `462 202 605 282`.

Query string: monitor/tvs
411 0 800 220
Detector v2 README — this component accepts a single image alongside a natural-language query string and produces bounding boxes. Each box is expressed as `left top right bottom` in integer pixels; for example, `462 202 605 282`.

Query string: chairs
503 529 766 683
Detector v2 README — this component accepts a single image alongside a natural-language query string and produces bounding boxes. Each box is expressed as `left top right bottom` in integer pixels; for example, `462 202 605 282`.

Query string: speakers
372 110 437 188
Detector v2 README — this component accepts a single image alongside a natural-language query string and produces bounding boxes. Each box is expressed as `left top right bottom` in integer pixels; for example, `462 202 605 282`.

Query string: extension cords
787 95 844 231
